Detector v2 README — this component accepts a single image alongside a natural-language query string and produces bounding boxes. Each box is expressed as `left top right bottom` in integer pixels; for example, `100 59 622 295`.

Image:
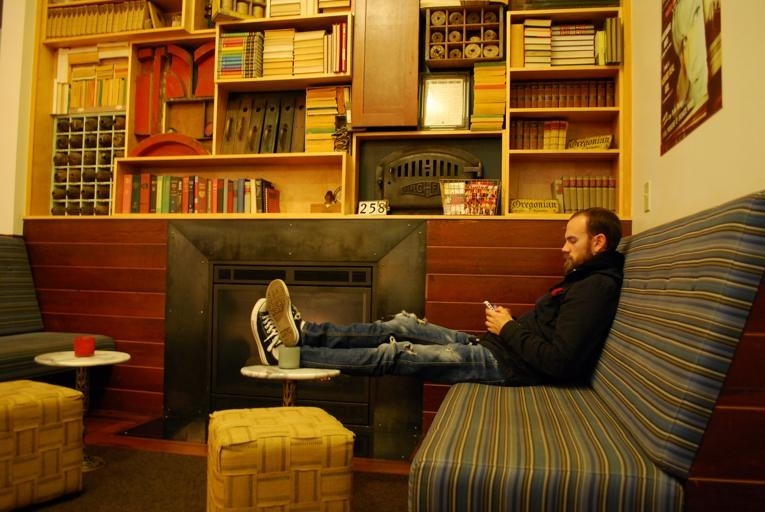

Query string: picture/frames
418 70 470 132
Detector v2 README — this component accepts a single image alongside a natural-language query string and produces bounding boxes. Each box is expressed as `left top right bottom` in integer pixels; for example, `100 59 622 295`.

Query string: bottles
50 116 127 216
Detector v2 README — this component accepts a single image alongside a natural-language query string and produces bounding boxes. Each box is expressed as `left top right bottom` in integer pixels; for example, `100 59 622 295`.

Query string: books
219 0 351 79
510 19 622 212
122 173 280 214
470 61 506 131
305 87 352 153
46 0 183 115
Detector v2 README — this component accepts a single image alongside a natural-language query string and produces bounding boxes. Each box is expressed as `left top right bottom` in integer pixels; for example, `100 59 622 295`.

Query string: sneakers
250 279 304 365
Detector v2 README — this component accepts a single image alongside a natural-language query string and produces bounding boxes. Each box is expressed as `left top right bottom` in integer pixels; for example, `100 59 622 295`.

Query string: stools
0 379 84 512
206 405 355 512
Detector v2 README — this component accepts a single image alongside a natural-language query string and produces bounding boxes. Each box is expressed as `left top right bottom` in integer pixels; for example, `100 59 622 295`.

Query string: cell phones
482 301 494 310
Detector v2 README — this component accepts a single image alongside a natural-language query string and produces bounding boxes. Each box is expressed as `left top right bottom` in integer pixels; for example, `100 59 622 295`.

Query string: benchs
0 234 117 382
406 194 763 509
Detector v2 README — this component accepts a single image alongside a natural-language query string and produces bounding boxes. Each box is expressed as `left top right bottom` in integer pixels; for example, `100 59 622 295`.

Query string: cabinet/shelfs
22 0 636 218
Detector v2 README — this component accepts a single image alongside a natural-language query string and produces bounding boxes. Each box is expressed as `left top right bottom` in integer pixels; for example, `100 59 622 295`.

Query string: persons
250 208 624 387
668 0 714 108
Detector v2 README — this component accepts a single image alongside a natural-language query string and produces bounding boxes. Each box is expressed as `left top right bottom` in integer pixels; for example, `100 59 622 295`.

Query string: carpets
13 443 407 510
